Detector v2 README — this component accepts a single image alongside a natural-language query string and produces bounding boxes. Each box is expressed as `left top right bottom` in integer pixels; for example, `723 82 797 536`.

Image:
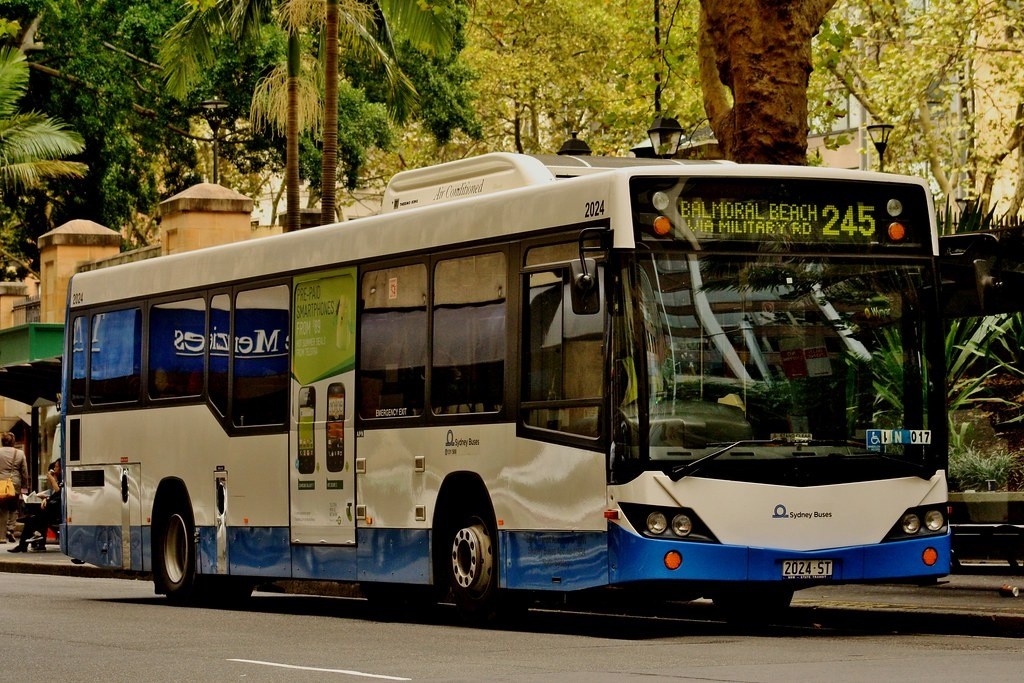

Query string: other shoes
0 538 6 543
26 535 43 542
31 546 46 550
7 546 27 553
5 531 16 543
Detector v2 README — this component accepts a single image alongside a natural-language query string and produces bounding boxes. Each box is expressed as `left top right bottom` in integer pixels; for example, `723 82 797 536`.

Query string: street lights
867 123 894 172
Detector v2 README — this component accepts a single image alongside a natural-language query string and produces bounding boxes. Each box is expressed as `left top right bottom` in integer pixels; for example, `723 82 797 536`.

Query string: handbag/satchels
0 477 16 498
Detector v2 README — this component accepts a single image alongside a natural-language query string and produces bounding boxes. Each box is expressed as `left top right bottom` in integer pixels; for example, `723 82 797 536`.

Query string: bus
60 153 979 614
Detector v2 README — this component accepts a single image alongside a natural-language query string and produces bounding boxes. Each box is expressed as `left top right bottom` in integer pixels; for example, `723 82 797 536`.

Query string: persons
0 431 29 544
7 462 56 553
25 458 61 552
